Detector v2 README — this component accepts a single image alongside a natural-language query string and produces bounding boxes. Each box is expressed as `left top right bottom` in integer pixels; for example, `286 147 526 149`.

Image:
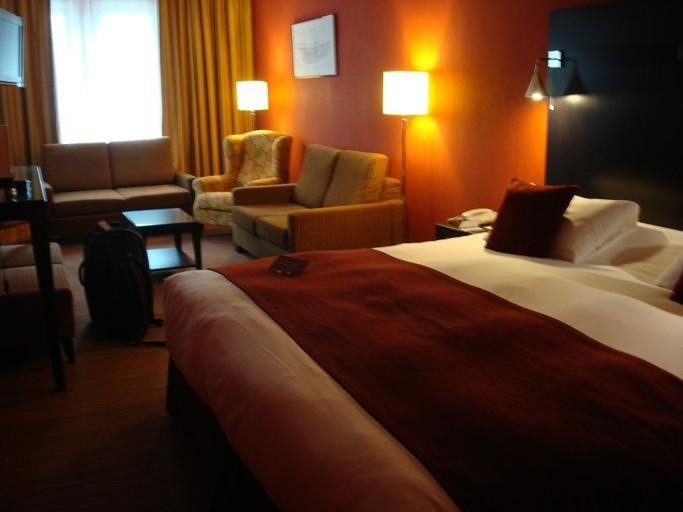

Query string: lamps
236 80 269 131
382 71 429 201
523 58 548 100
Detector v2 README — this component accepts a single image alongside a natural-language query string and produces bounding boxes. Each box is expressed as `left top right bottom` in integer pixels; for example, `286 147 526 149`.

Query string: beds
161 0 683 512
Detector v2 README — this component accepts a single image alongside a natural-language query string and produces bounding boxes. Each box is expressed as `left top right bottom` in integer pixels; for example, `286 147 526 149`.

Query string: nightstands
435 221 496 240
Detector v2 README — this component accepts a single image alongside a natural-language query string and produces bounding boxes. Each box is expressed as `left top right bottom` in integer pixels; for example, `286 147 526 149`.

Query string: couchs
192 129 292 235
0 242 76 363
231 144 405 258
42 136 197 244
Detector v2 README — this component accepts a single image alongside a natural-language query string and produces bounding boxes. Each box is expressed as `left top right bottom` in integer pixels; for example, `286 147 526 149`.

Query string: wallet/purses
271 255 304 276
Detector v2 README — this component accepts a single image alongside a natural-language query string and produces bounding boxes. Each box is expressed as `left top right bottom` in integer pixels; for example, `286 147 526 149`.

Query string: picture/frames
289 13 339 79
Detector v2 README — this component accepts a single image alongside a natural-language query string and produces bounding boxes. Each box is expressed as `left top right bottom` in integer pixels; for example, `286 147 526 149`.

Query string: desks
1 165 65 393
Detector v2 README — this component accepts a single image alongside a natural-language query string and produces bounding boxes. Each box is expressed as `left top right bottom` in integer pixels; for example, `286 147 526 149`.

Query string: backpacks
79 221 166 347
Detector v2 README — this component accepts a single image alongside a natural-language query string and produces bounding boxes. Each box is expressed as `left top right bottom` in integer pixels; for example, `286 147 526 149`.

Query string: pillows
485 177 579 258
655 252 683 289
669 272 683 304
41 135 175 193
293 144 390 207
528 182 640 263
